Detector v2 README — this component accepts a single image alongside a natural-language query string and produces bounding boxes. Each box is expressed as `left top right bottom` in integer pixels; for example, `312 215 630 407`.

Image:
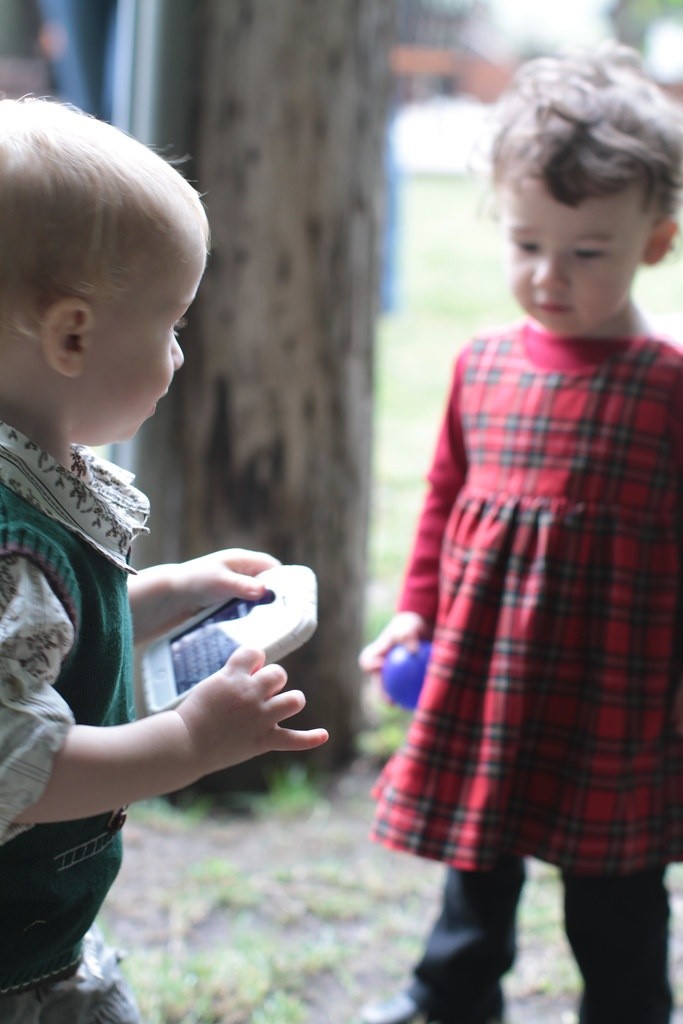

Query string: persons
0 99 328 1024
356 60 683 1024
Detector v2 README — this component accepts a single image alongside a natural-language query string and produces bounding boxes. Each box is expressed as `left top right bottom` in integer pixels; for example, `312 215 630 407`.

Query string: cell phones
141 564 318 715
